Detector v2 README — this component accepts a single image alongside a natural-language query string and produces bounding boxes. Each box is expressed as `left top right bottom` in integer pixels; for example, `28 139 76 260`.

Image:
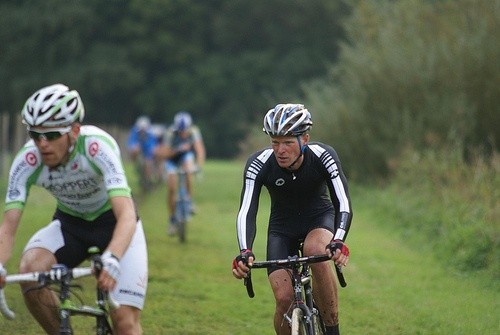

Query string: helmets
173 112 192 132
20 83 84 127
133 117 151 131
262 103 312 136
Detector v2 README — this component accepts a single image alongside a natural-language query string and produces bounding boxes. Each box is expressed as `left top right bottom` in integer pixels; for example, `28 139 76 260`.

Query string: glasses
26 126 71 141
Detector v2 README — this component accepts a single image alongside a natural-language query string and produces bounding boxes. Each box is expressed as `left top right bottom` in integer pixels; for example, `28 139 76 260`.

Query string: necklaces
292 172 297 181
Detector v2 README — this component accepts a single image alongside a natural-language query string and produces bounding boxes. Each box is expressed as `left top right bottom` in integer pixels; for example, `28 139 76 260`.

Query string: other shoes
188 205 197 215
169 224 178 235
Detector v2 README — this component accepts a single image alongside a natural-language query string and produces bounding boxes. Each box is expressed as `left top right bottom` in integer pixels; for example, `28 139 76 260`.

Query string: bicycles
0 246 114 335
166 169 202 242
240 244 347 335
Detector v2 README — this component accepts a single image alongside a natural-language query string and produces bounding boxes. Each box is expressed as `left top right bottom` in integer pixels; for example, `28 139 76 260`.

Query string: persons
158 111 207 236
127 116 161 190
0 82 149 335
231 102 354 335
